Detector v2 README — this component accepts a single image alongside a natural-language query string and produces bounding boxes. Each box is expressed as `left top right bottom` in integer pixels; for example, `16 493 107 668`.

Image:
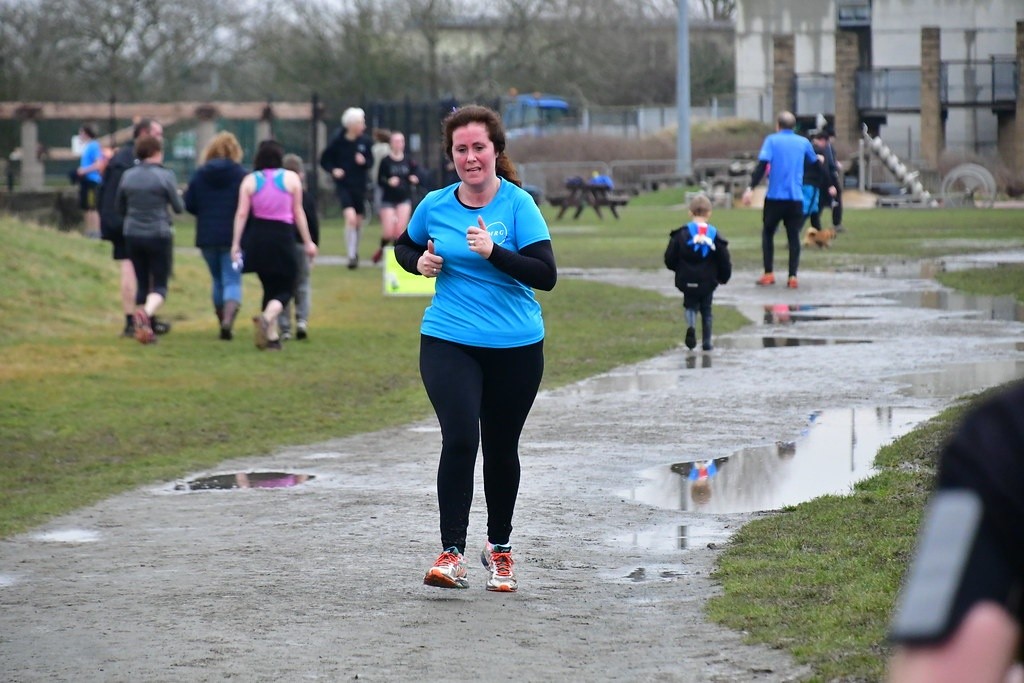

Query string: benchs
595 197 630 206
548 192 571 206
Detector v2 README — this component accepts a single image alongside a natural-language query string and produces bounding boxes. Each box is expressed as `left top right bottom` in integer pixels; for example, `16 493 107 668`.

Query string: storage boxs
383 242 437 296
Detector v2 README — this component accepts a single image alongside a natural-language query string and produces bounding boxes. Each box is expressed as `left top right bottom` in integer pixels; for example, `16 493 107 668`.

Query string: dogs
803 226 836 252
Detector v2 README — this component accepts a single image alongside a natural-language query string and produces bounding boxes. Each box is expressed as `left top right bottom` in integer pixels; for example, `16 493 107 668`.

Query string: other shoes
220 327 232 341
295 320 308 339
252 316 271 352
755 276 774 285
269 339 281 351
788 279 799 289
125 326 136 339
134 308 156 344
685 327 697 350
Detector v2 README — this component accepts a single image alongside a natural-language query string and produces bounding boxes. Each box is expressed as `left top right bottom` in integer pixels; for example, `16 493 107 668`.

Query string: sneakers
480 540 518 591
421 546 469 590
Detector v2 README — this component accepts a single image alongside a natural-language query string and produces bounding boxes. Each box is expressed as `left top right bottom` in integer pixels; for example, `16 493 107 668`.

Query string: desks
556 181 620 220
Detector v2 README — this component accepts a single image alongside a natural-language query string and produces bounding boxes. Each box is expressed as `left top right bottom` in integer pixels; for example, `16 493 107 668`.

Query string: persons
277 152 320 342
441 154 461 188
883 377 1024 683
665 195 732 349
182 129 250 340
799 125 846 245
113 138 185 343
672 457 728 504
742 110 836 289
321 107 374 270
587 169 614 194
98 118 169 336
230 141 318 351
372 130 434 264
392 105 558 591
69 123 118 240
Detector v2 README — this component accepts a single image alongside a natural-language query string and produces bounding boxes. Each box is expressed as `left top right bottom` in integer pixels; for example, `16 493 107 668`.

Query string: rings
433 268 437 274
472 240 475 246
